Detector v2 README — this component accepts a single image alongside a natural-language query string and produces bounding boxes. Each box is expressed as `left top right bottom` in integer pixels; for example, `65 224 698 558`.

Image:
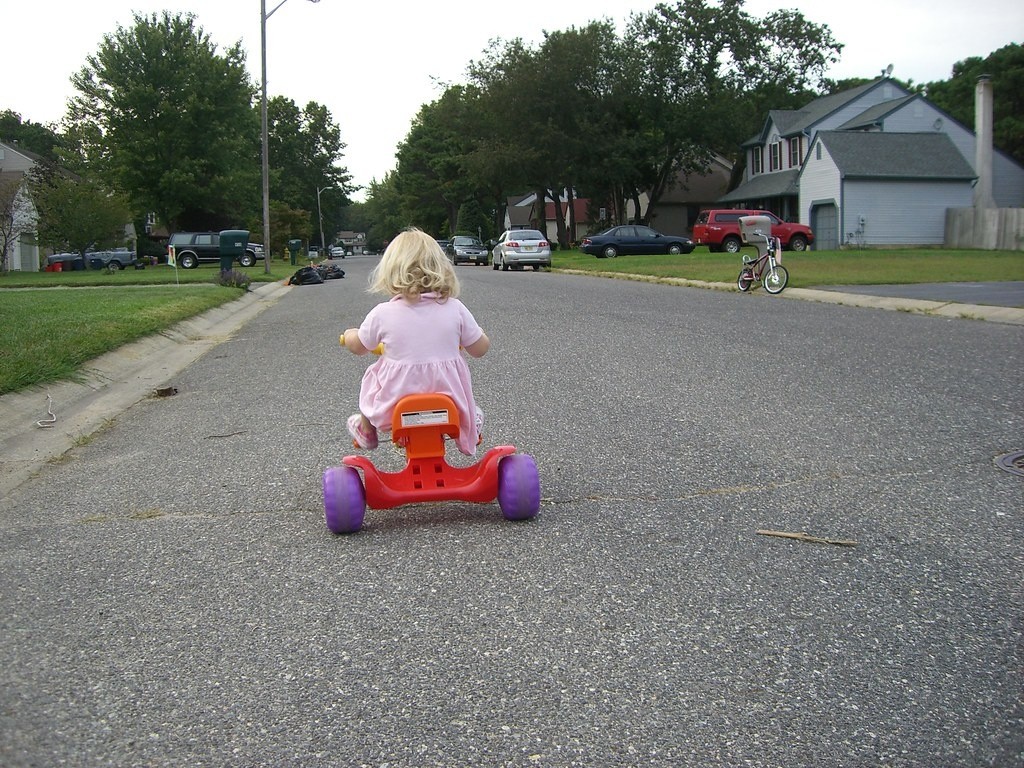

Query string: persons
344 226 489 456
328 242 334 259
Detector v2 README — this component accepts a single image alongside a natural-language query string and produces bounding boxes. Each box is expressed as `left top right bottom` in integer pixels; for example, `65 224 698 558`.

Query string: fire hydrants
283 246 291 260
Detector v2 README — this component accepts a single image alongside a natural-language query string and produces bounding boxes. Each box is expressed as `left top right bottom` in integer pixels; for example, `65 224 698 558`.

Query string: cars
579 225 696 259
47 243 137 271
435 239 452 254
491 229 552 272
444 235 489 267
331 247 346 259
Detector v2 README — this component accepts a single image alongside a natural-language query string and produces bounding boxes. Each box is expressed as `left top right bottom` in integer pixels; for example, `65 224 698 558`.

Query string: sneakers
475 406 484 445
347 414 378 450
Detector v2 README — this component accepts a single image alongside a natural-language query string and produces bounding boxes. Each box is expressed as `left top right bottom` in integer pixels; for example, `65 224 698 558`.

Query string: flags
168 245 176 266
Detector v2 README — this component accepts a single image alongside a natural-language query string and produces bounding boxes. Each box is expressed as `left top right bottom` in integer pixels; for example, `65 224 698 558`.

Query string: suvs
167 232 272 269
692 208 814 253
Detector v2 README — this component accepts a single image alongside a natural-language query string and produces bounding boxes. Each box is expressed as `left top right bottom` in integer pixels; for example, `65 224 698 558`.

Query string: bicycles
736 232 788 294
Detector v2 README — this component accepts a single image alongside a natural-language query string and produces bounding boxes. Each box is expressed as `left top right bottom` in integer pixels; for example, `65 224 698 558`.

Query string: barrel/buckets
53 263 63 272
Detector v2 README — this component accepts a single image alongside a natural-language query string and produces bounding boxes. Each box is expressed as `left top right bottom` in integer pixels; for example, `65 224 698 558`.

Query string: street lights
258 1 322 274
317 186 334 257
